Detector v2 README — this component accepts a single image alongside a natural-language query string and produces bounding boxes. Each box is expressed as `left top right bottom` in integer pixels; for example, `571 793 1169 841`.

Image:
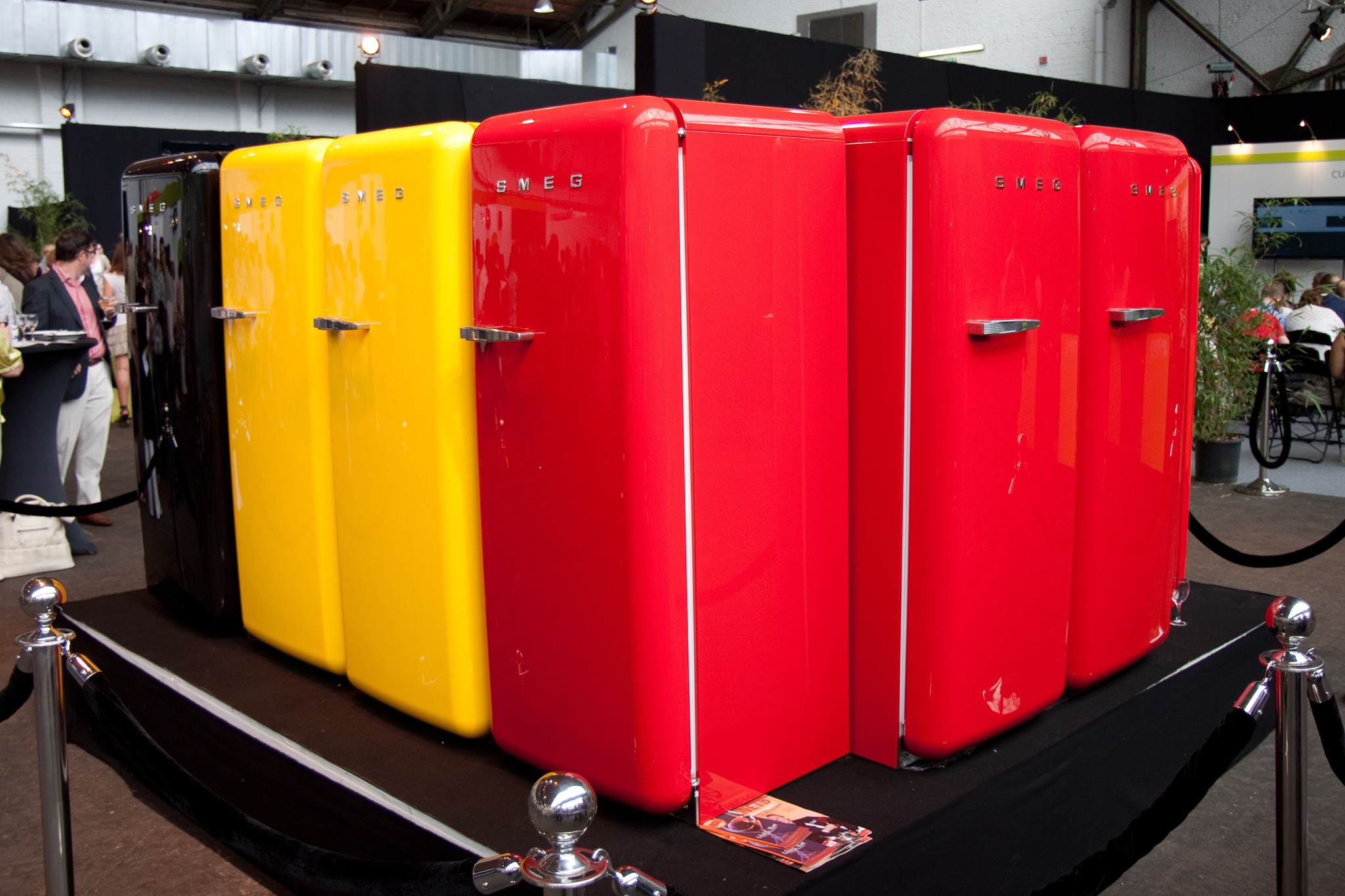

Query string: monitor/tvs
1252 196 1345 261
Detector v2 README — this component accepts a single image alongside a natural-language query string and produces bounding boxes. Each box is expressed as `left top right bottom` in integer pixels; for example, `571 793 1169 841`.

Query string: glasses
85 250 96 256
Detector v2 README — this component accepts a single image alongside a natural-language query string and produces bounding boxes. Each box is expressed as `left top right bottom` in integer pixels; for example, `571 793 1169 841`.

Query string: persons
0 225 132 557
766 815 860 844
124 218 180 438
1231 271 1345 406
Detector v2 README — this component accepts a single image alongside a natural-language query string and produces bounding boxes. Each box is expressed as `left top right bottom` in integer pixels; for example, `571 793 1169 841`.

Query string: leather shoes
78 512 114 525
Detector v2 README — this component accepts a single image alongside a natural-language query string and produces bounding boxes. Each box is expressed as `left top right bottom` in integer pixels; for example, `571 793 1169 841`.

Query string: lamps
533 0 554 13
60 104 75 123
1309 22 1334 42
244 54 271 76
361 37 381 64
145 45 172 66
1299 120 1317 140
1227 125 1244 144
308 61 332 80
68 38 95 61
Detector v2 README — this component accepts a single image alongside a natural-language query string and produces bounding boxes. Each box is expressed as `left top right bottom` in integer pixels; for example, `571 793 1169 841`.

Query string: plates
43 331 88 341
24 330 69 341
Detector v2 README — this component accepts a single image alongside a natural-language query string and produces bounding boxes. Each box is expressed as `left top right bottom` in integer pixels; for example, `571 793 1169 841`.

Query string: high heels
118 405 129 427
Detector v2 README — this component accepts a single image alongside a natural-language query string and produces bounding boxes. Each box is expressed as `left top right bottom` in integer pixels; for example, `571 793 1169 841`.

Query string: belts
88 358 104 368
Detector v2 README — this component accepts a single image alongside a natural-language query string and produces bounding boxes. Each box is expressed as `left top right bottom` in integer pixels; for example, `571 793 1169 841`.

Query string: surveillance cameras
244 53 270 74
145 43 170 67
67 37 94 59
308 60 332 79
1215 61 1235 75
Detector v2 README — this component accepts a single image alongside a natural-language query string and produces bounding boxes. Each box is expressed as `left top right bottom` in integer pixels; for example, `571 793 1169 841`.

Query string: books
698 794 873 873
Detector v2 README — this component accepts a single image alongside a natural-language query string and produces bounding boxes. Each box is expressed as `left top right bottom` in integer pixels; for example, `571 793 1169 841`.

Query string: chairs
1270 331 1345 464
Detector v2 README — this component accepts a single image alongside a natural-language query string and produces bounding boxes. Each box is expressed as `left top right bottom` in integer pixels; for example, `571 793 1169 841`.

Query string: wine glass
99 296 114 322
14 314 27 342
25 313 39 342
1169 577 1190 626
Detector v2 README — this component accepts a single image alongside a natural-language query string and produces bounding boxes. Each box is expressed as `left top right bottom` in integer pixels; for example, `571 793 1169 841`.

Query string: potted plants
1192 197 1336 483
